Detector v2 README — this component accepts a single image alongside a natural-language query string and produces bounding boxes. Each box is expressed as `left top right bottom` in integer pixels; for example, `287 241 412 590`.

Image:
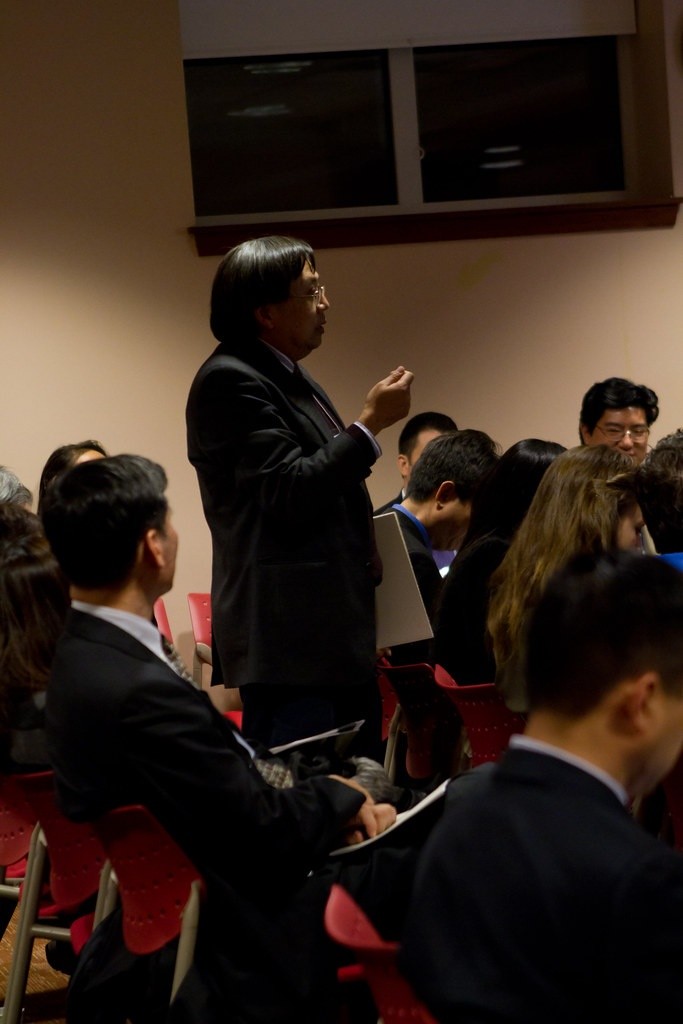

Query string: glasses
590 421 649 441
263 286 325 306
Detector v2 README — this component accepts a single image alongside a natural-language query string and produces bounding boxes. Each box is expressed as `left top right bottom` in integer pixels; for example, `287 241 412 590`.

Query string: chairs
1 594 531 1024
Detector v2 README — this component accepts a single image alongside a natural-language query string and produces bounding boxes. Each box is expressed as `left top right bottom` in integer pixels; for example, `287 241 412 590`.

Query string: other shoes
45 935 80 977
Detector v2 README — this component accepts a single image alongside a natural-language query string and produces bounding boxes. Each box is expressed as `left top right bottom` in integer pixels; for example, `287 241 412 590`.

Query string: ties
160 633 293 789
295 362 344 439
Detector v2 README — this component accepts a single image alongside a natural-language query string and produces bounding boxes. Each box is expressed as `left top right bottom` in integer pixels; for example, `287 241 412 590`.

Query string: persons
185 236 415 756
410 546 683 1024
0 442 398 1024
372 376 683 714
